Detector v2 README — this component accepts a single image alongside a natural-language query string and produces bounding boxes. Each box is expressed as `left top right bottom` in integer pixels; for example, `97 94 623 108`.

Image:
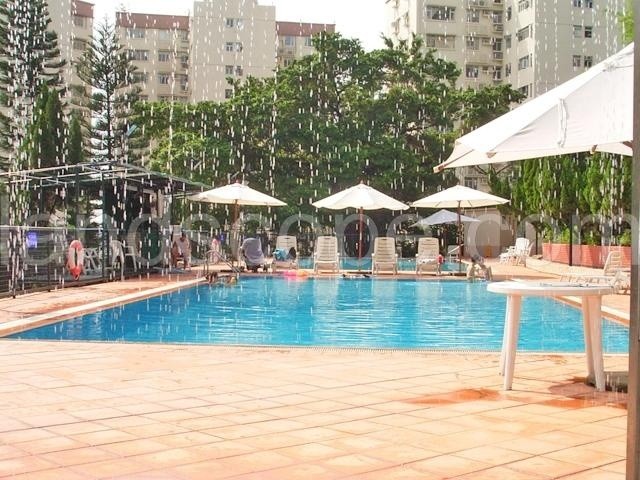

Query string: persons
466 255 483 282
172 232 191 270
239 231 264 272
475 257 492 281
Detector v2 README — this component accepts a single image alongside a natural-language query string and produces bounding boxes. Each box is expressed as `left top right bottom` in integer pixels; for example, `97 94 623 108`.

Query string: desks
486 282 614 392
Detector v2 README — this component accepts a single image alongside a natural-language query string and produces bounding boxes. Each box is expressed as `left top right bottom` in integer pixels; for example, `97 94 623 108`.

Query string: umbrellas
408 209 480 255
312 180 410 269
410 184 510 271
188 180 287 266
433 40 634 174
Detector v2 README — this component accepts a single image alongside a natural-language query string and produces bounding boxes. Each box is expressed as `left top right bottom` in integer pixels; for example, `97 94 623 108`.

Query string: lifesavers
69 239 84 276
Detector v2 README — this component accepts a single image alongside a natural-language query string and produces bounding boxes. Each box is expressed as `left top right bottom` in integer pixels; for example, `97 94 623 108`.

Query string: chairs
500 238 530 267
559 250 624 284
108 240 137 282
171 234 442 275
